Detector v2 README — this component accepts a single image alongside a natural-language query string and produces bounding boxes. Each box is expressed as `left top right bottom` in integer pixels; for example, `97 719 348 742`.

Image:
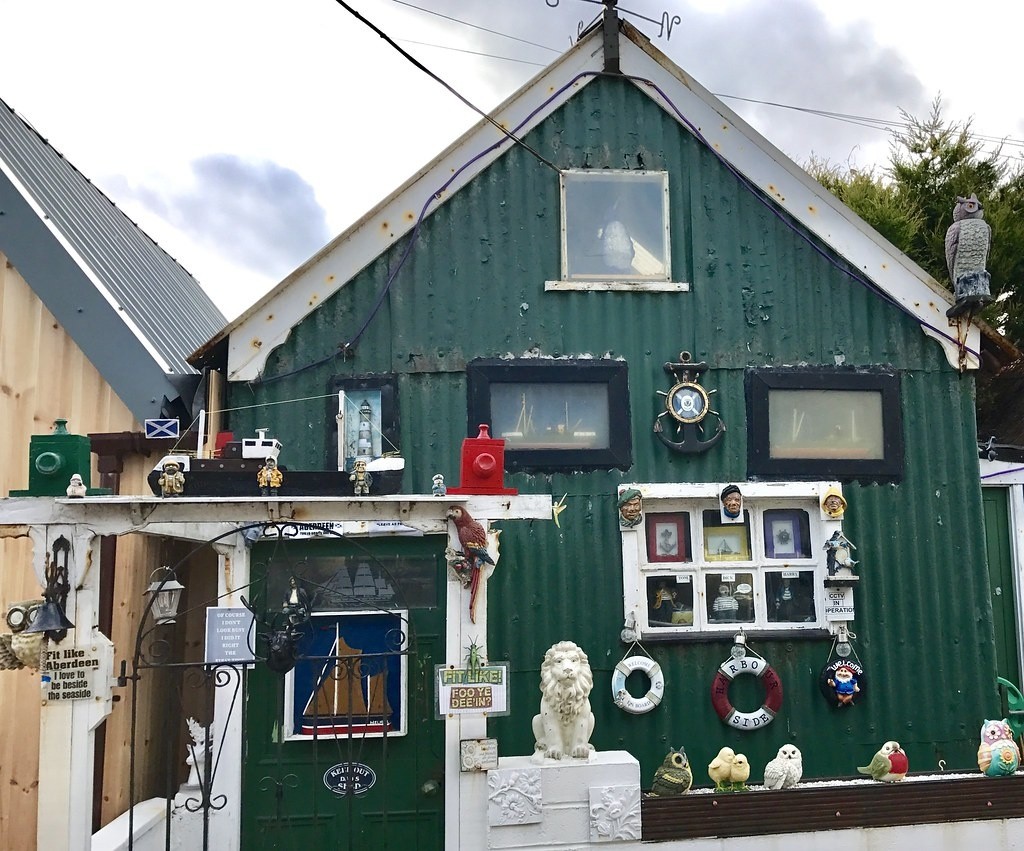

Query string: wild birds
856 740 908 782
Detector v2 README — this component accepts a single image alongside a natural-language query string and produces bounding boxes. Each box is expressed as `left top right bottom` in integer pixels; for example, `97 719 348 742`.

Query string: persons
617 487 642 527
432 474 447 497
349 460 373 496
158 461 185 497
821 489 847 518
66 474 87 498
721 485 741 519
257 456 283 496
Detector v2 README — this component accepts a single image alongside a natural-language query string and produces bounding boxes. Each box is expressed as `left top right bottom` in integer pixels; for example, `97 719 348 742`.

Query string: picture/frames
646 514 686 562
764 513 801 558
702 526 748 562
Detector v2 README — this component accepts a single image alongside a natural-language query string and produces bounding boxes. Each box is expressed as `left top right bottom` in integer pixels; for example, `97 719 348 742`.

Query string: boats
770 408 870 459
147 390 405 497
502 394 597 448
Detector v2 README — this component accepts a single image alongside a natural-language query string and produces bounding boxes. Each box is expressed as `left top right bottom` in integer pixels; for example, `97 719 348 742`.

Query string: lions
532 641 595 761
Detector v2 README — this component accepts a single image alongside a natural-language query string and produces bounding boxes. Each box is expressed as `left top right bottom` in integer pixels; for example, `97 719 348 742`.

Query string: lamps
140 565 184 625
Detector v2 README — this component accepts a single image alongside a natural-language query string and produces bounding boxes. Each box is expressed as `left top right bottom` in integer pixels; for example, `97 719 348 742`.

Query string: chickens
707 747 750 792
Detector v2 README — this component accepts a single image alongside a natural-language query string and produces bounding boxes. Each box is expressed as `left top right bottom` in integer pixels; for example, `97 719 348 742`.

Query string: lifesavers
611 656 665 715
712 658 782 732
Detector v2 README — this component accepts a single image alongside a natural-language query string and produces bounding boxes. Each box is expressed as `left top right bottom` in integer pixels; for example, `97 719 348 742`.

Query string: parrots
445 505 497 625
